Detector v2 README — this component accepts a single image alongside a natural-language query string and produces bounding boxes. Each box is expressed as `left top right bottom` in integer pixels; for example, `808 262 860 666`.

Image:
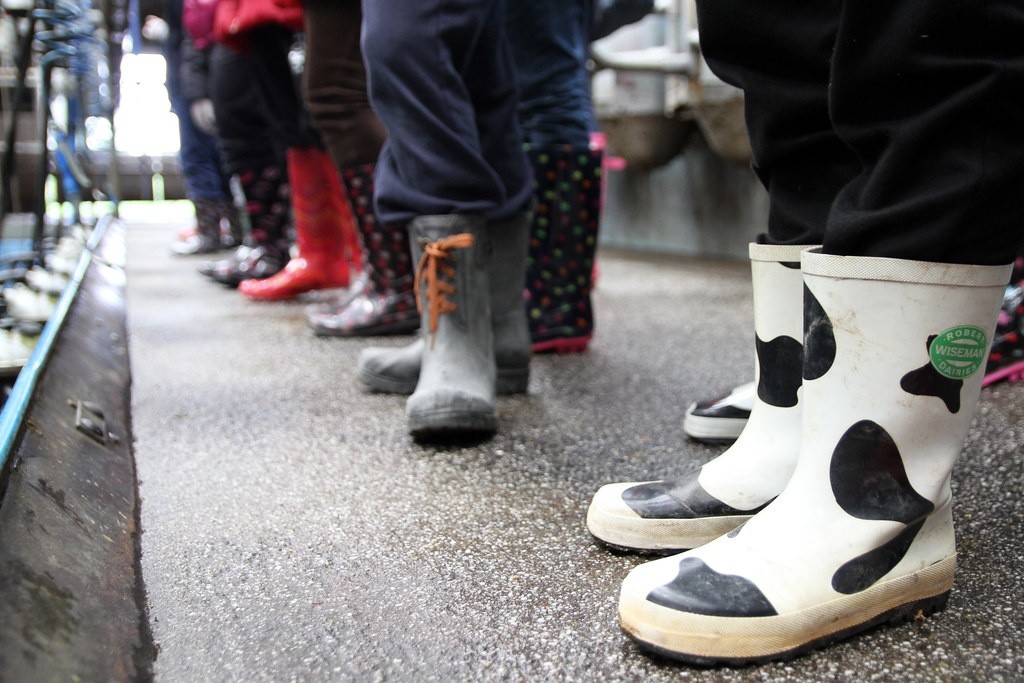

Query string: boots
520 145 608 353
586 242 1015 664
299 211 423 338
356 203 534 442
195 165 294 287
234 148 356 302
171 198 241 254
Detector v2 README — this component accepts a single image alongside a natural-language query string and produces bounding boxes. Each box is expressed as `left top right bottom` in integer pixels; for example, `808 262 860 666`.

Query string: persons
962 1 1022 386
130 1 664 445
586 1 1024 666
675 1 952 451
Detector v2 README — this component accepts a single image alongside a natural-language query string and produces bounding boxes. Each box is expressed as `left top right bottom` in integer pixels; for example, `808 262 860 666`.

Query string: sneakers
978 288 1024 390
680 376 759 447
